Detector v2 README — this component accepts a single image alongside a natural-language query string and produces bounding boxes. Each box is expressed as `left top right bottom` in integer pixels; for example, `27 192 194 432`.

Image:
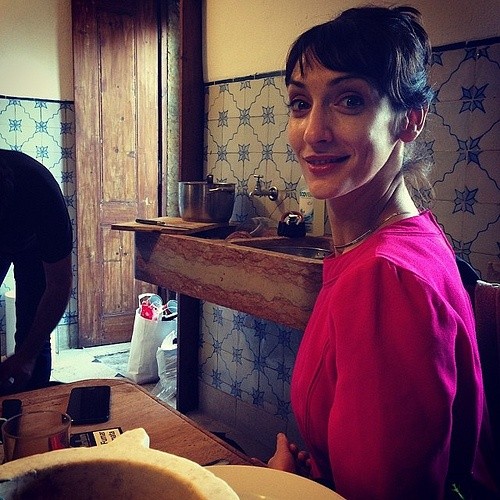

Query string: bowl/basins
1 443 239 500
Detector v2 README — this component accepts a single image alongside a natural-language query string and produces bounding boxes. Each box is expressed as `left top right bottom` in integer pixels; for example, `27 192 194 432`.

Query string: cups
1 410 72 462
2 398 23 419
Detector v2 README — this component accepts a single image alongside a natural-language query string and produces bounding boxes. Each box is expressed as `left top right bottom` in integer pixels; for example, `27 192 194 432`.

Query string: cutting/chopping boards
111 216 229 234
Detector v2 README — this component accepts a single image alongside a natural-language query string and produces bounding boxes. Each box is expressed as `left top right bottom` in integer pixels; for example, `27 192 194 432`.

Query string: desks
0 378 268 467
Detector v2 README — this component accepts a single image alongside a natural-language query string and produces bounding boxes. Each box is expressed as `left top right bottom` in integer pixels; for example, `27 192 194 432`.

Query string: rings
8 377 15 384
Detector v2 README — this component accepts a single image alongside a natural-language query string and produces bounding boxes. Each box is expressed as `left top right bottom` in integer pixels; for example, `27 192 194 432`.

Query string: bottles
140 300 154 321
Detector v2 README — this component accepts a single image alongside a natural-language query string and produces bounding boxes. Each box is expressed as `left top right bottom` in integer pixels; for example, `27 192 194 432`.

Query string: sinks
133 217 288 297
231 233 336 330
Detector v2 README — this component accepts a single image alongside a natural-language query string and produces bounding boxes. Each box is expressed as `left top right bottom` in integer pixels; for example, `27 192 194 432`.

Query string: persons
0 149 74 395
267 7 493 500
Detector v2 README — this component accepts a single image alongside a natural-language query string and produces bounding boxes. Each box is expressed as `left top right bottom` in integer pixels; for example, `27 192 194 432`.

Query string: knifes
135 219 193 230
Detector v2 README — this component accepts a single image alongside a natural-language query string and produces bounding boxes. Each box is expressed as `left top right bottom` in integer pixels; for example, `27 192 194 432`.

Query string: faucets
248 174 277 198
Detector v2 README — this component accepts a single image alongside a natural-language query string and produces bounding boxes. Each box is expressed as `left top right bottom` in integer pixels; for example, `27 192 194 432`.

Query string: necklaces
333 211 418 249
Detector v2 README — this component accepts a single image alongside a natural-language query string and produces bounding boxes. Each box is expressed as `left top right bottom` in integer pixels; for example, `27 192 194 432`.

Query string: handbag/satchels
125 293 178 385
155 329 178 397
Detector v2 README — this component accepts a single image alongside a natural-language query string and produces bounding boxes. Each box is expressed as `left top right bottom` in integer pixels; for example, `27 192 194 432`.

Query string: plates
201 464 348 500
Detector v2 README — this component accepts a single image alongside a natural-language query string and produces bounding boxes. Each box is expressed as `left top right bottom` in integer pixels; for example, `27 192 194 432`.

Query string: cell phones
69 426 123 448
66 385 111 425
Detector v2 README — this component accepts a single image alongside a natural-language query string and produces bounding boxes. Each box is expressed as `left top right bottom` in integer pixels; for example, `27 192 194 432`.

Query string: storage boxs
68 427 123 448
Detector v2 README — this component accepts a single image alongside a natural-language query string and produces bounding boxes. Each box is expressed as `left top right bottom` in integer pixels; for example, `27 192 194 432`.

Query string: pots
177 181 234 223
